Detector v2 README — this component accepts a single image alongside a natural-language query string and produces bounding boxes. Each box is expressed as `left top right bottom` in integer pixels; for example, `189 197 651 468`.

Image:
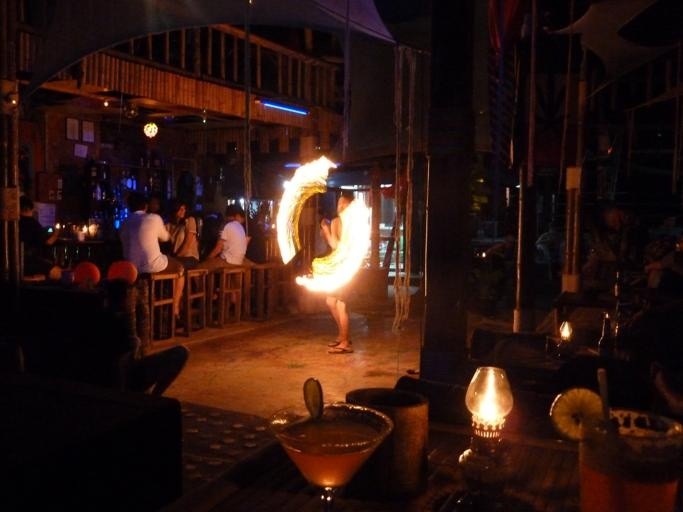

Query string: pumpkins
108 260 139 286
71 262 102 290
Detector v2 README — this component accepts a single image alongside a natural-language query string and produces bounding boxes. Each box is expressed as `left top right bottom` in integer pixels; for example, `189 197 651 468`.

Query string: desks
154 401 681 511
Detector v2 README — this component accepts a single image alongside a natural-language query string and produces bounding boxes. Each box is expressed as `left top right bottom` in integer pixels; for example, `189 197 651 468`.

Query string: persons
318 191 353 355
462 200 683 428
17 187 266 322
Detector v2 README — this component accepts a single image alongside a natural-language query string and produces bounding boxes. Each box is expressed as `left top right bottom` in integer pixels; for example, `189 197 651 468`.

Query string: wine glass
268 400 396 512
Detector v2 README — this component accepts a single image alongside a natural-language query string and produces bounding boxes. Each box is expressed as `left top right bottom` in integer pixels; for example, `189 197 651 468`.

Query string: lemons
303 379 325 420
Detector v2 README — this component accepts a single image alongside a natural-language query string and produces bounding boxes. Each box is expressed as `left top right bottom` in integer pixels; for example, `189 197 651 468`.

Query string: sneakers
168 314 187 328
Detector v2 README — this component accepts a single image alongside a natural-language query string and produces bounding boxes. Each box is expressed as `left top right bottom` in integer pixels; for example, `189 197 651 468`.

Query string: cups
578 406 683 512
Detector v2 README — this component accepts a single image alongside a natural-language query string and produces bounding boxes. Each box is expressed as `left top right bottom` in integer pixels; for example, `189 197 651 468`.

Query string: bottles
50 244 94 266
597 313 616 360
614 271 623 297
87 164 162 240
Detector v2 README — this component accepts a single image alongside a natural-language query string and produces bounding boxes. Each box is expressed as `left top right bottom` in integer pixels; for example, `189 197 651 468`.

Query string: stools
140 260 291 344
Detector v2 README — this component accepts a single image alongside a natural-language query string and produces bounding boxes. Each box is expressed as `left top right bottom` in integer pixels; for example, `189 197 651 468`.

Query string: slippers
328 341 352 347
328 348 353 354
